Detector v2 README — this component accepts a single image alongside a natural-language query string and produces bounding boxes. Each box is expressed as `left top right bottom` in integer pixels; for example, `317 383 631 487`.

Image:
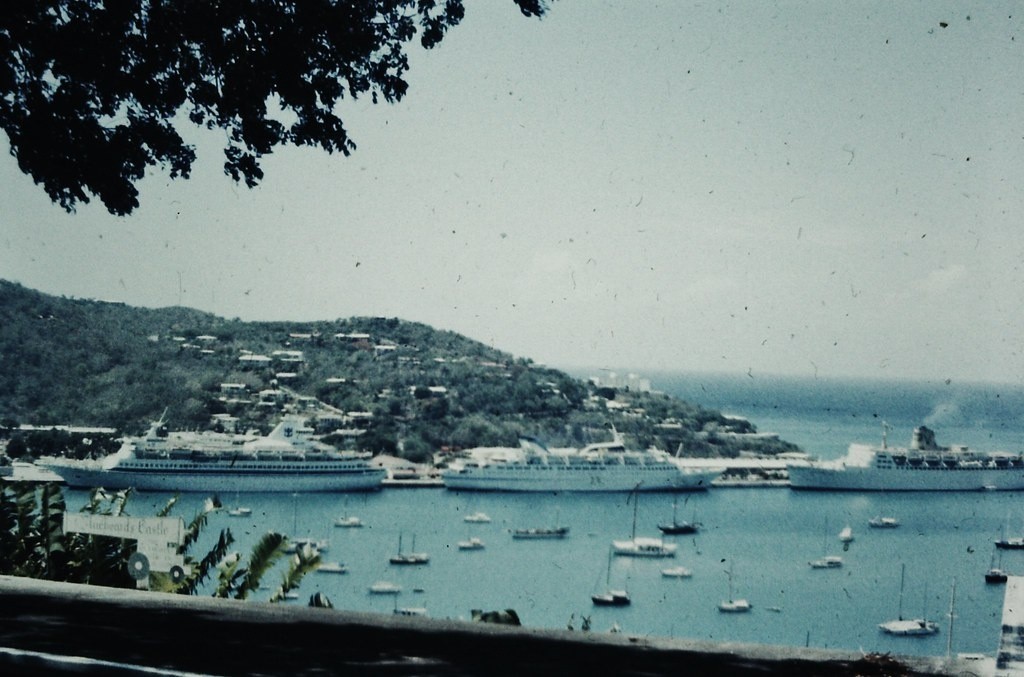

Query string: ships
32 406 388 495
786 420 1024 496
435 436 728 491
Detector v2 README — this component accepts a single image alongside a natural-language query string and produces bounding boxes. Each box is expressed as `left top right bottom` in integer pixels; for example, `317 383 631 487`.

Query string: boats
591 589 631 605
808 557 844 570
197 496 329 600
367 582 402 594
657 498 703 534
868 515 900 528
334 514 363 527
389 530 429 565
458 539 485 550
661 567 694 579
984 566 1008 583
879 619 941 635
393 606 430 619
991 535 1024 549
718 598 752 614
507 527 572 540
464 512 491 524
314 563 350 574
610 537 679 557
838 526 854 543
